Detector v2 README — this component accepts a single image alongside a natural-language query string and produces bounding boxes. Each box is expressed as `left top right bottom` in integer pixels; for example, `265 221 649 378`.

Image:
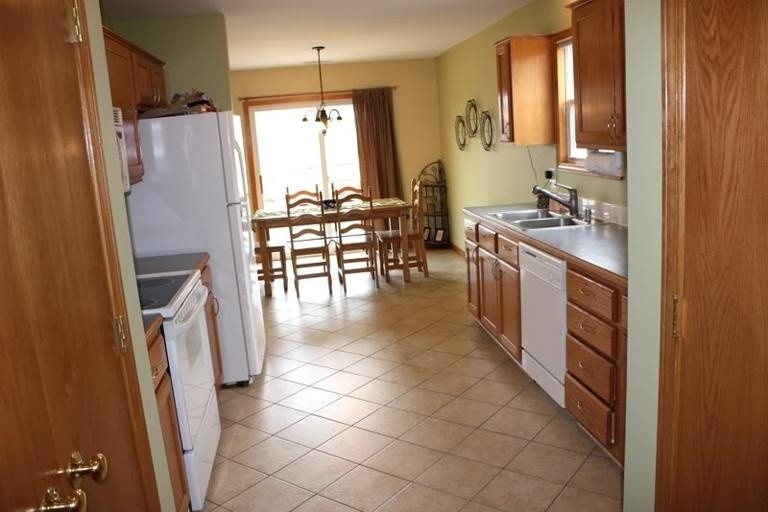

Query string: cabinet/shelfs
480 217 522 367
564 0 627 150
464 208 479 322
417 160 451 250
144 314 192 512
564 256 627 466
102 24 169 186
494 35 556 146
199 252 225 396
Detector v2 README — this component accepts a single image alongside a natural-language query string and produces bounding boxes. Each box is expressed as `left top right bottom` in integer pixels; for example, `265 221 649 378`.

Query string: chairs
254 179 429 297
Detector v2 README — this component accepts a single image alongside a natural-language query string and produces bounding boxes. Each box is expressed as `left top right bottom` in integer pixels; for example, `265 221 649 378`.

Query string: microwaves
112 107 131 194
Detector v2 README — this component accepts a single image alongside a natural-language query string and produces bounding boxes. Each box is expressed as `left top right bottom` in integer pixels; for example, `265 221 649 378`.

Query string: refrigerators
126 112 267 387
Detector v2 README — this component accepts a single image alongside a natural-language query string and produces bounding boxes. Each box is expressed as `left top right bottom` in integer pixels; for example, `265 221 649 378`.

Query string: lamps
302 45 343 137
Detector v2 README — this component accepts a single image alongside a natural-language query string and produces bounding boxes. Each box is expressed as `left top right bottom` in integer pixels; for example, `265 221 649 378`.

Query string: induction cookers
134 270 201 316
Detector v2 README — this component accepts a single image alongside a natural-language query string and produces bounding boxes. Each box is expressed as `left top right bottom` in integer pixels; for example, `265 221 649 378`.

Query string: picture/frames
433 228 446 243
423 226 431 241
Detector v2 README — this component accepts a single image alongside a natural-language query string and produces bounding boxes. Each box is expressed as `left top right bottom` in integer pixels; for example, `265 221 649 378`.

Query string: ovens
165 280 223 509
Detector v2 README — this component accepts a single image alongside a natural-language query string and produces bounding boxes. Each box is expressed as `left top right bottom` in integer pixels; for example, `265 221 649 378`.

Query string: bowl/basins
320 201 341 209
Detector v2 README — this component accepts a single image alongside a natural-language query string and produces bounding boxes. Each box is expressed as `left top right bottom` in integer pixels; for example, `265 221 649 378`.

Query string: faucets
532 183 578 216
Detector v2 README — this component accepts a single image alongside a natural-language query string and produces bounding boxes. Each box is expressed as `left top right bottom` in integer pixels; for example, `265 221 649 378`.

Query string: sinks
519 217 586 229
490 210 561 221
